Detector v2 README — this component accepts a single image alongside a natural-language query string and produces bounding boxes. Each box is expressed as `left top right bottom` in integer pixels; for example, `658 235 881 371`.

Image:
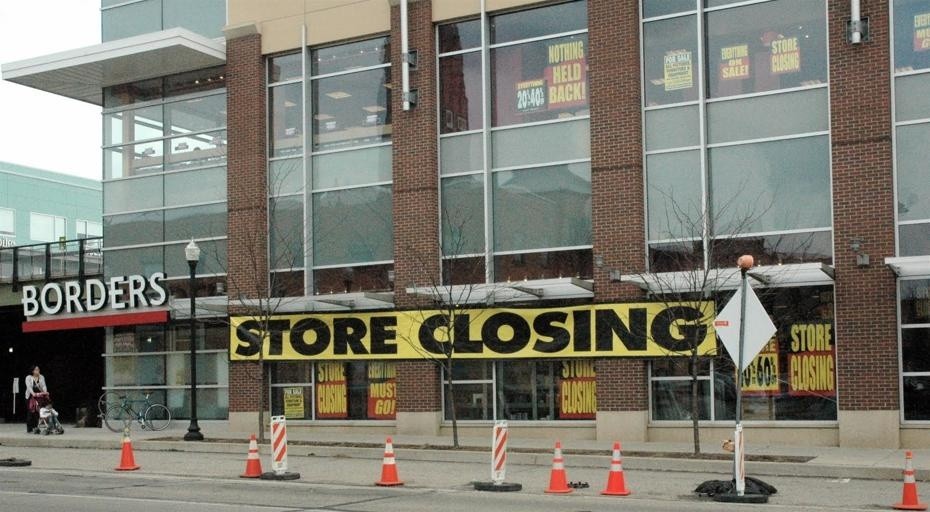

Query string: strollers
32 392 65 436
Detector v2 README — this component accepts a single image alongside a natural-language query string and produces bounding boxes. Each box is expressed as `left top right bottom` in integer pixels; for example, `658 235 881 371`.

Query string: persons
23 362 47 434
39 401 64 434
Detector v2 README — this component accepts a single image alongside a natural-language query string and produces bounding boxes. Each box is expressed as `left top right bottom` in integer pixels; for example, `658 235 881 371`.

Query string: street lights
182 236 205 442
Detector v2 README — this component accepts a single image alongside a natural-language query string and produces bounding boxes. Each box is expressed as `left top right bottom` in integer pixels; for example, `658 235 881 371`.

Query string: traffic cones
240 434 265 478
892 448 928 511
113 430 141 471
602 444 630 497
374 438 404 485
543 441 575 494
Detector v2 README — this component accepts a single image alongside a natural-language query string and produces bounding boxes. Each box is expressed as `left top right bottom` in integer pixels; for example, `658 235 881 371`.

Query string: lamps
851 243 870 266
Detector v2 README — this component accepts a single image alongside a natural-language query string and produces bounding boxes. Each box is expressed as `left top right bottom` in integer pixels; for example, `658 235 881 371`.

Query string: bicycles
99 388 173 433
